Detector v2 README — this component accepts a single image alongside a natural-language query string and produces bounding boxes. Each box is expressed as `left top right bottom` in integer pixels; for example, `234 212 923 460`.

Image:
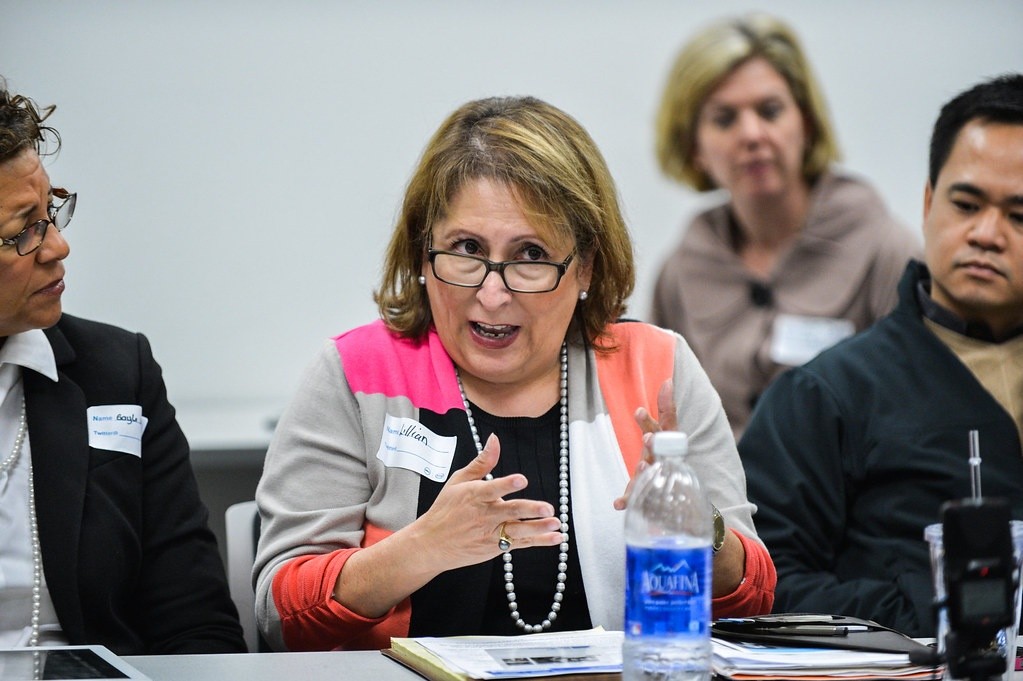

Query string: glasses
0 187 77 257
426 223 582 293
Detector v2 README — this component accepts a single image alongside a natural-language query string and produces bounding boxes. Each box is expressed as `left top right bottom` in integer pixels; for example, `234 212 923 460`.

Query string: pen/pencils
745 626 850 638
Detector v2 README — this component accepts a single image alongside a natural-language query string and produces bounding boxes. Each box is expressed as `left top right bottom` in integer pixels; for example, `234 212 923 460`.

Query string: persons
0 17 1023 657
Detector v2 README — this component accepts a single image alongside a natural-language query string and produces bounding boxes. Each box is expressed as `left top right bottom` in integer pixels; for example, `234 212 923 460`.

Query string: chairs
226 501 259 653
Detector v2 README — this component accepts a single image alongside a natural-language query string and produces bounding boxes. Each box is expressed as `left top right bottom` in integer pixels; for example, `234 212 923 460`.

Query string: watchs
711 503 724 557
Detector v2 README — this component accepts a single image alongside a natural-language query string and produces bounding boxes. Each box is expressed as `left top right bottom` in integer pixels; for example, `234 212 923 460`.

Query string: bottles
623 430 712 681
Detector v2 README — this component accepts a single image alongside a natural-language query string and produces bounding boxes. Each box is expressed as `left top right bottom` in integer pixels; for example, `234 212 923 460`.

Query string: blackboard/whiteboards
0 0 1023 474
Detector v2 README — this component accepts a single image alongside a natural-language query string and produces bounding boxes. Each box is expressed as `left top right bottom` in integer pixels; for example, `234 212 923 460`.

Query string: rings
498 522 514 551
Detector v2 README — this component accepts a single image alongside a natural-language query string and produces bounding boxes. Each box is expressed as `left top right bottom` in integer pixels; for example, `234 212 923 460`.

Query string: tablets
0 645 154 681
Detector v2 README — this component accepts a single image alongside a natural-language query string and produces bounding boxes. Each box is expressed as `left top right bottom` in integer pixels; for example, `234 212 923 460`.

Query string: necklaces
453 340 568 632
0 388 26 469
33 653 42 680
30 466 41 646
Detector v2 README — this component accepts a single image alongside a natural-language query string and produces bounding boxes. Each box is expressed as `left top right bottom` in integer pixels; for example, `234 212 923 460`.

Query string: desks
176 397 298 466
117 650 426 681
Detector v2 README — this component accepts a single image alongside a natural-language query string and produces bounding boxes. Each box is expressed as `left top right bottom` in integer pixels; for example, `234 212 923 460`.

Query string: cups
924 518 1023 681
